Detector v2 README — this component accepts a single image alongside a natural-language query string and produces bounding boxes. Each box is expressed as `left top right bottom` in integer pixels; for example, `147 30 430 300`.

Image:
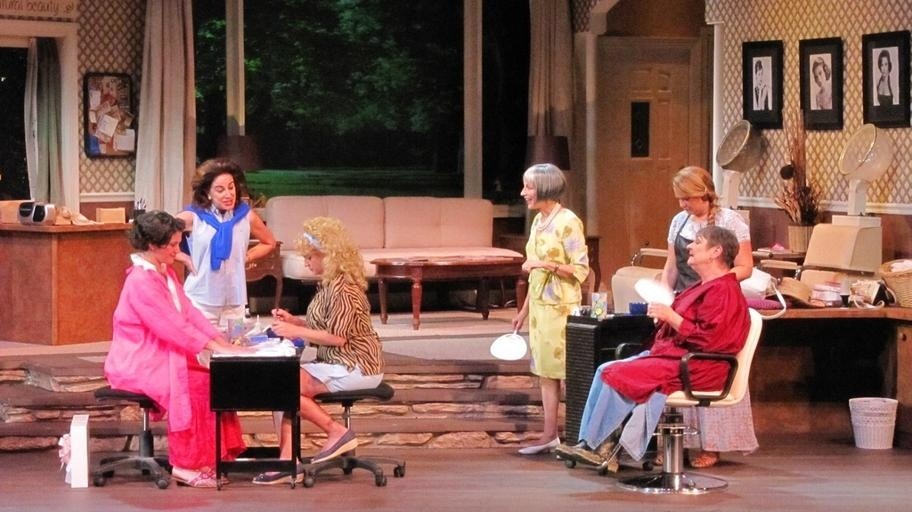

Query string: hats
768 274 827 310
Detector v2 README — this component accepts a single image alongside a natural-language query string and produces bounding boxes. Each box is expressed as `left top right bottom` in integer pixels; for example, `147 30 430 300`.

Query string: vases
787 223 814 253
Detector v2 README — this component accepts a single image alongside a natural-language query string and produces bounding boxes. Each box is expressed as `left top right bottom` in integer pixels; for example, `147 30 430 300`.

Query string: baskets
877 257 912 309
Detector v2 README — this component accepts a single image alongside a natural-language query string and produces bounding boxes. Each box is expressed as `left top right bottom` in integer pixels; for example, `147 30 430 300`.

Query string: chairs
610 249 672 313
757 223 882 279
556 305 763 496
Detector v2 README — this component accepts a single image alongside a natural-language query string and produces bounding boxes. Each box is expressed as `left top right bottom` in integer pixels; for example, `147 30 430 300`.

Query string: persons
808 56 831 109
105 210 254 489
752 60 770 112
250 217 385 487
488 162 590 455
169 158 275 318
651 167 753 469
553 225 751 475
873 50 900 106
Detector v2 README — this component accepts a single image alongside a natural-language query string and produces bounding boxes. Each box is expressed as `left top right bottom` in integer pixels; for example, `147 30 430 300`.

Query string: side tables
245 239 283 309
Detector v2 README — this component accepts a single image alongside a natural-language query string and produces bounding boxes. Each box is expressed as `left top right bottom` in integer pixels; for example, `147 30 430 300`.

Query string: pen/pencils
135 199 147 209
275 306 279 317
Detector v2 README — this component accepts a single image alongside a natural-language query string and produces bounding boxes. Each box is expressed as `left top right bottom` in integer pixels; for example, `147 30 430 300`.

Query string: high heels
518 437 561 455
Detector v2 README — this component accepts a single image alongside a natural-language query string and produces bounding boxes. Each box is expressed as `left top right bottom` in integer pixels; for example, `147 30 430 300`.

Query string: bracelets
554 262 559 275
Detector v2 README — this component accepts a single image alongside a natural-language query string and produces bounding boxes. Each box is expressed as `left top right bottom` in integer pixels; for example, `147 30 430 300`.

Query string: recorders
17 202 55 226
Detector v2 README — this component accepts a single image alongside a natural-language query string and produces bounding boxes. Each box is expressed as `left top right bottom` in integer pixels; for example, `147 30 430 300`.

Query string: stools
94 383 175 490
303 380 407 487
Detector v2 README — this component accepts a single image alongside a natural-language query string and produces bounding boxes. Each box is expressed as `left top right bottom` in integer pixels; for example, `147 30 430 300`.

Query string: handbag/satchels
737 268 788 320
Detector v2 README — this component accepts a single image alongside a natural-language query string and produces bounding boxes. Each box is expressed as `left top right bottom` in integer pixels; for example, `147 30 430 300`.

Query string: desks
758 309 912 451
210 338 300 489
0 221 187 346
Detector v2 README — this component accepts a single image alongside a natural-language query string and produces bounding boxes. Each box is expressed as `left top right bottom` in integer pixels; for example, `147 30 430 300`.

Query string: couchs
263 194 524 308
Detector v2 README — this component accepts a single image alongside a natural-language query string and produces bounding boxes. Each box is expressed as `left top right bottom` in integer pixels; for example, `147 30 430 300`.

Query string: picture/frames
741 39 782 129
799 36 843 130
83 70 134 157
861 29 910 129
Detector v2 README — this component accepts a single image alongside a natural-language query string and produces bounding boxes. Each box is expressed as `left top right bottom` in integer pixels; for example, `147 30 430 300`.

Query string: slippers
172 466 230 488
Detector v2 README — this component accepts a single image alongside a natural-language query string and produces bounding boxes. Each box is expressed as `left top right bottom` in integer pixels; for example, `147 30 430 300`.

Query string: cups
629 301 648 313
134 209 146 221
226 316 258 347
590 292 606 320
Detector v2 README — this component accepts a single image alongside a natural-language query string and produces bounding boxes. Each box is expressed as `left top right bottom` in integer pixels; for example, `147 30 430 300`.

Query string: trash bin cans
848 397 899 449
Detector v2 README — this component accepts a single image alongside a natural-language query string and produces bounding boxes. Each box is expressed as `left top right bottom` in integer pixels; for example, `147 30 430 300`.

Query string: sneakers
252 463 304 484
572 446 620 474
555 443 575 459
311 429 358 464
